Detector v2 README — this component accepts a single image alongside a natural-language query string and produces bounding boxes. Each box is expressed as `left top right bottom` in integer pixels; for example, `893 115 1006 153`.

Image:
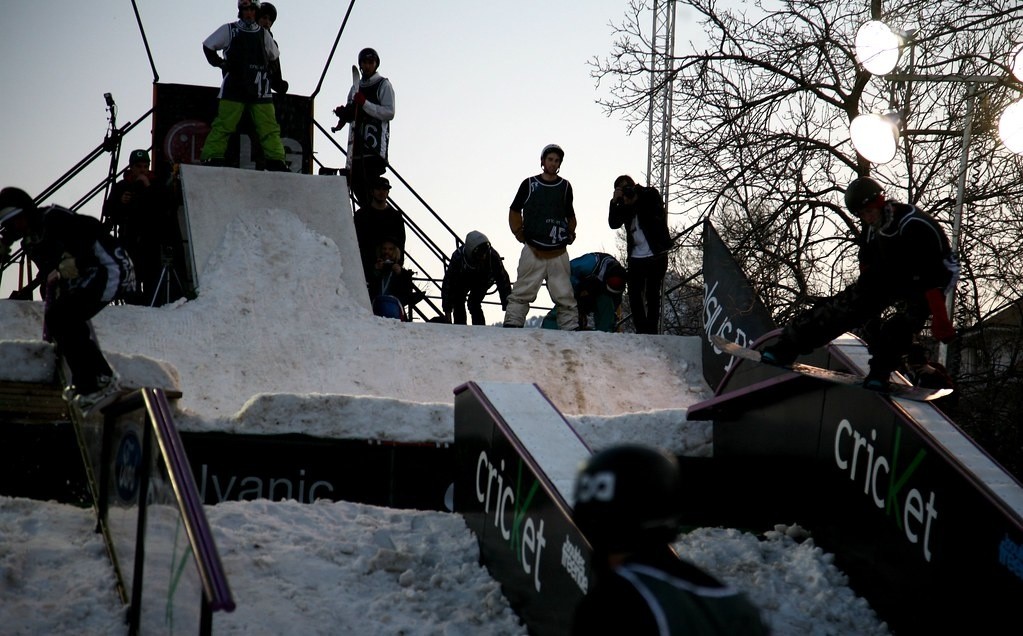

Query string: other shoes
762 338 798 371
862 359 894 390
202 158 223 167
68 387 121 418
273 160 291 173
62 374 122 400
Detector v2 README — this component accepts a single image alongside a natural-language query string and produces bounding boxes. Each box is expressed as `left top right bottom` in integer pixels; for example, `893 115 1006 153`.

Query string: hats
908 344 929 365
605 266 626 289
372 178 392 190
130 149 149 162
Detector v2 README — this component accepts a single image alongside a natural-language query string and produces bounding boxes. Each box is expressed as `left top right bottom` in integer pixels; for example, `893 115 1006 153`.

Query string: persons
609 176 674 335
542 253 627 332
0 187 123 418
365 240 414 322
197 0 293 172
902 342 954 390
570 446 776 636
103 149 184 307
353 177 405 284
332 48 394 207
502 148 579 331
760 177 955 398
442 233 512 326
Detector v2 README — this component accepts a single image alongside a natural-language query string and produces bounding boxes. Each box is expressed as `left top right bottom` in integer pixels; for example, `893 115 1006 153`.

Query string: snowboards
346 65 360 193
61 384 122 421
710 333 955 402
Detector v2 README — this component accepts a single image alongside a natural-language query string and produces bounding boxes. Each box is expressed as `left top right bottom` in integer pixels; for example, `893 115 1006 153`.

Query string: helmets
256 3 276 23
541 144 565 166
238 0 260 9
359 48 380 66
0 207 23 225
473 243 491 255
845 177 882 212
574 445 687 542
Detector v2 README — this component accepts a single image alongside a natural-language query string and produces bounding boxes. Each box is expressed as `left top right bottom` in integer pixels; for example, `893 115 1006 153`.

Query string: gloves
216 58 232 71
926 290 958 342
860 262 868 272
272 80 288 94
355 93 366 105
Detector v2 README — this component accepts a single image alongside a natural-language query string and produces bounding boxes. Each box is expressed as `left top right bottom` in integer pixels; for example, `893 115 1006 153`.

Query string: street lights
851 21 1023 383
